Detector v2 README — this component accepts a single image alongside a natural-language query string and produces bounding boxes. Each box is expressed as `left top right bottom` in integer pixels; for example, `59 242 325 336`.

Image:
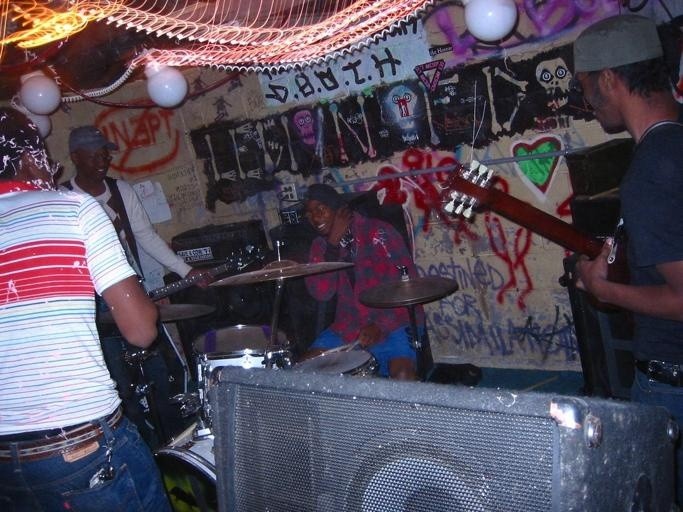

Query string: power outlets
280 183 299 201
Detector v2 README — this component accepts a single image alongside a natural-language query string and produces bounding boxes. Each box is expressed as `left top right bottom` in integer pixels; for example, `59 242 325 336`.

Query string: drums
189 324 288 407
285 349 379 378
151 419 216 508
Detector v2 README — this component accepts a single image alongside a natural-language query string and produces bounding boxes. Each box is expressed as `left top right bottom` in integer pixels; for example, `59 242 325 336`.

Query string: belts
634 356 682 389
0 403 124 463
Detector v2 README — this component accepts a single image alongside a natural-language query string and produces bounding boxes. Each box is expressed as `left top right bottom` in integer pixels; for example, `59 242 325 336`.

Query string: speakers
568 186 634 243
566 138 635 195
562 255 635 396
163 190 434 383
209 365 680 512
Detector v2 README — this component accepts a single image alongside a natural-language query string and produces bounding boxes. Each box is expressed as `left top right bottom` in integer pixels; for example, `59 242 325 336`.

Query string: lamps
12 37 62 117
6 100 53 141
460 1 520 44
133 42 188 110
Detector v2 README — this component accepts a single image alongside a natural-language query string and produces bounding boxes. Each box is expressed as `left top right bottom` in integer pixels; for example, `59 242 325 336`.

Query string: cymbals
207 259 356 286
360 277 459 308
153 302 218 323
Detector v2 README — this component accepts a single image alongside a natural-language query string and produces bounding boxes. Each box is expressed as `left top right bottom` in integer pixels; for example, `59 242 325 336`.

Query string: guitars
96 243 264 334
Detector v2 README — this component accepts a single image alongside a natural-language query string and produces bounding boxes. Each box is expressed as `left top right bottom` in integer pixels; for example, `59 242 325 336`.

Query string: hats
572 13 664 74
68 125 117 151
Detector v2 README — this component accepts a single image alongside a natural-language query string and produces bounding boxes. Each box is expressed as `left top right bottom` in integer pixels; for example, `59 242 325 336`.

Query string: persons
300 183 426 381
574 13 683 512
0 105 177 511
59 126 201 431
